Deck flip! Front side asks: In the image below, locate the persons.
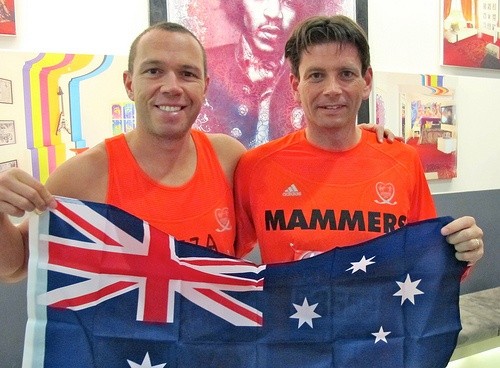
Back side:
[234,14,484,281]
[0,23,248,285]
[192,0,342,150]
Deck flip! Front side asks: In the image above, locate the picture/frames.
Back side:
[148,0,369,150]
[438,0,500,72]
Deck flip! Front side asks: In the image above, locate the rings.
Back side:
[475,238,479,248]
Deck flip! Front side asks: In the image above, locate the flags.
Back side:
[22,196,469,368]
